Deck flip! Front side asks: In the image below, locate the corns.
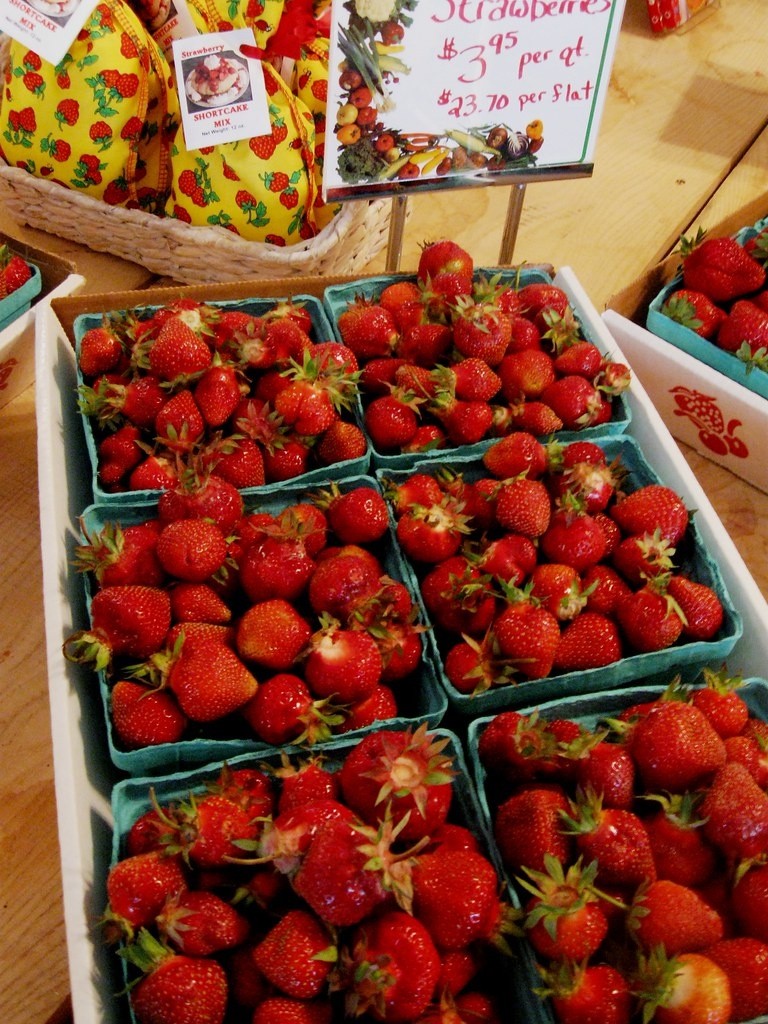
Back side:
[338,54,411,74]
[446,130,501,156]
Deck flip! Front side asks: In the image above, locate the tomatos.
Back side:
[436,158,453,175]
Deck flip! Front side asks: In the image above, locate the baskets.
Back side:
[0,136,392,284]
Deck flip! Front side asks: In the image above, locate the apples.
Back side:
[376,134,394,152]
[397,163,420,179]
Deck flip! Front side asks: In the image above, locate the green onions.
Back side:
[336,17,395,113]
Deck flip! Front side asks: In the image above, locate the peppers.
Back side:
[487,155,506,170]
[526,120,544,154]
[335,21,404,145]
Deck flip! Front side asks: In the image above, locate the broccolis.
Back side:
[342,0,418,39]
[336,137,377,184]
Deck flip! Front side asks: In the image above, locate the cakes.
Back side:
[186,53,248,104]
[28,0,78,15]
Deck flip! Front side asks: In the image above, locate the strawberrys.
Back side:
[0,241,32,301]
[71,225,768,1024]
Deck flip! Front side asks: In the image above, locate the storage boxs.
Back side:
[1,193,768,1024]
